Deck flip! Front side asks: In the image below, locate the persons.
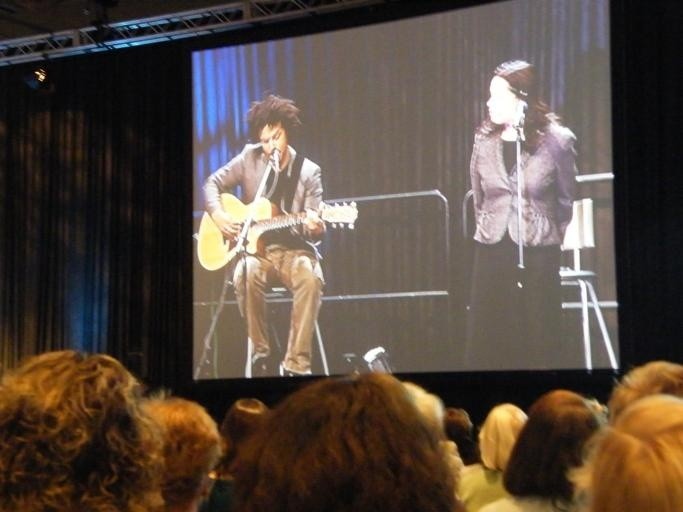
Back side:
[459,57,578,370]
[446,362,683,511]
[0,347,470,512]
[199,92,327,377]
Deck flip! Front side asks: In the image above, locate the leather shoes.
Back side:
[251,353,301,377]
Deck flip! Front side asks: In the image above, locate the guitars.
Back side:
[198,193,358,271]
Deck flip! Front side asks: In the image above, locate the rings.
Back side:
[224,224,231,232]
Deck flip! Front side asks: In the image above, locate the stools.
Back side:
[558,271,618,369]
[245,284,331,378]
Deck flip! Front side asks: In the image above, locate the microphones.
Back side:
[268,141,281,162]
[512,100,529,126]
